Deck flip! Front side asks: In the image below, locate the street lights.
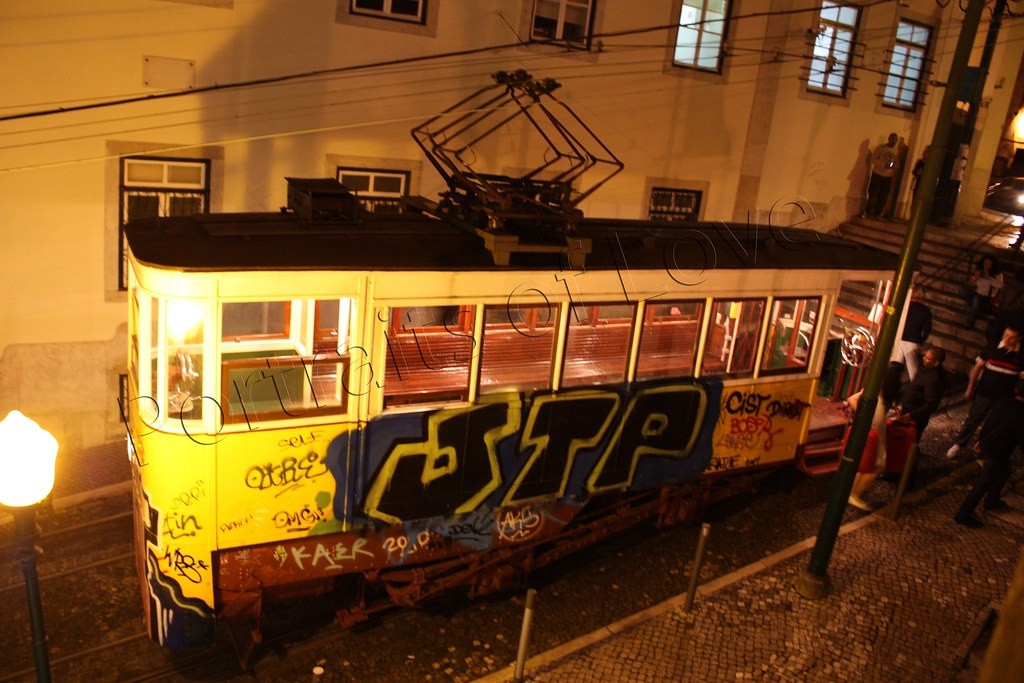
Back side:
[1,411,63,682]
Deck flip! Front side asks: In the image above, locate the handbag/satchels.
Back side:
[884,419,916,474]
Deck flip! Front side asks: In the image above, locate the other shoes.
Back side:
[984,499,1007,511]
[848,495,872,512]
[946,443,961,459]
[954,512,985,529]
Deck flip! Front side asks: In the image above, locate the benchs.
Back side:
[384,318,726,395]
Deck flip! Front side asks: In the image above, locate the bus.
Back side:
[116,170,926,678]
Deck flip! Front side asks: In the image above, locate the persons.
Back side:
[911,145,930,223]
[947,254,1024,525]
[1008,223,1024,250]
[843,284,946,513]
[861,133,899,221]
